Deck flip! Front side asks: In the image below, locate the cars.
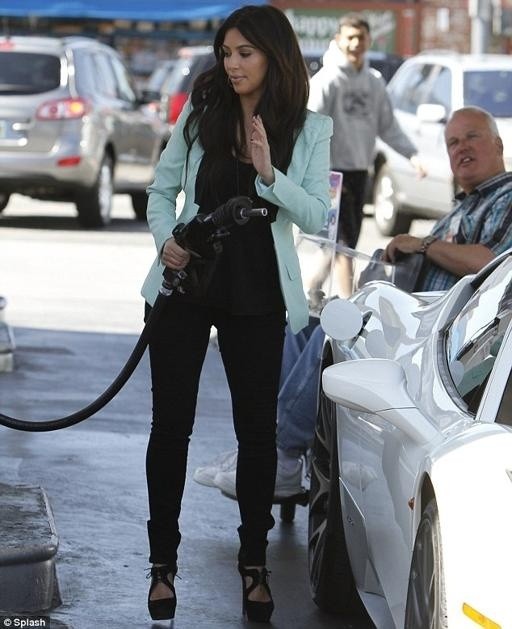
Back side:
[372,48,512,237]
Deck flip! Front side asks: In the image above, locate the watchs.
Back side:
[419,235,437,253]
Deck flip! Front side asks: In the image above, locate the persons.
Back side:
[139,5,333,623]
[308,12,429,313]
[193,107,510,504]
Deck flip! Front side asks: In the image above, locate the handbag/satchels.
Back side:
[357,249,423,293]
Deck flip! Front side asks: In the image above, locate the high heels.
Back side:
[147,559,179,620]
[237,561,275,621]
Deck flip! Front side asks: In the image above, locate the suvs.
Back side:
[159,45,217,125]
[0,33,173,226]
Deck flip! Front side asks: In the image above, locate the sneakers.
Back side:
[214,451,301,499]
[193,448,241,486]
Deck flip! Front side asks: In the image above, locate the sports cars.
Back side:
[304,247,512,629]
